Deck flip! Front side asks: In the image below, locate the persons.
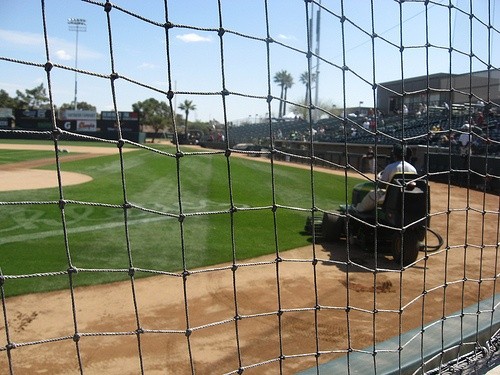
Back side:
[177,81,500,217]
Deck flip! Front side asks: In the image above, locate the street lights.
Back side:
[67,17,86,109]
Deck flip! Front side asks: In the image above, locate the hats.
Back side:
[391,143,407,159]
